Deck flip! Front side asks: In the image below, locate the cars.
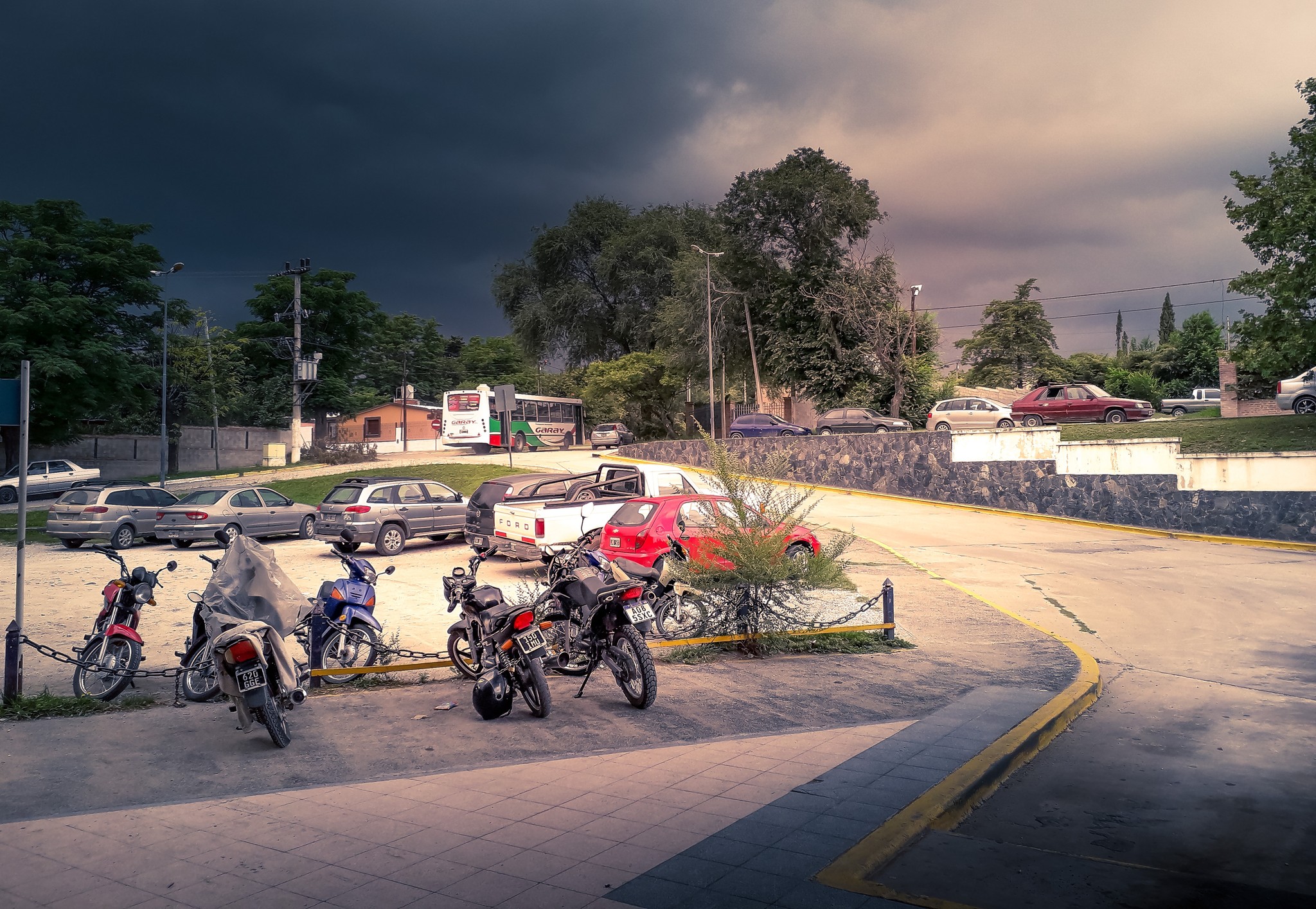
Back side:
[1276,364,1316,415]
[816,408,913,436]
[44,484,186,549]
[0,458,106,502]
[598,494,823,585]
[925,397,1019,431]
[591,423,635,451]
[1011,383,1156,427]
[317,477,479,558]
[728,414,813,438]
[460,471,600,559]
[153,488,320,546]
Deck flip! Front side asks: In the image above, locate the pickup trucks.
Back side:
[493,461,759,558]
[1162,387,1224,418]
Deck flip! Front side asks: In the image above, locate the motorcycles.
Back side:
[291,550,398,685]
[191,525,315,747]
[610,521,711,641]
[524,531,663,713]
[440,544,553,722]
[69,542,177,701]
[177,550,247,703]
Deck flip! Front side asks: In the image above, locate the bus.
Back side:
[439,388,588,448]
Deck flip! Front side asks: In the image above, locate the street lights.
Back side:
[151,261,187,482]
[690,243,725,439]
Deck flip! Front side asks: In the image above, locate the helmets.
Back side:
[472,667,518,721]
